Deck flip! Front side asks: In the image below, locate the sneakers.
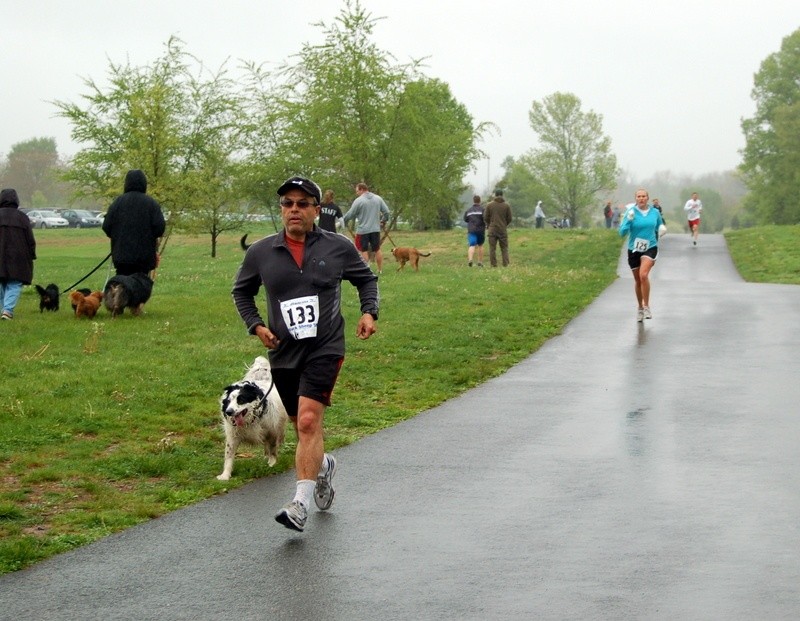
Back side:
[313,454,338,510]
[275,499,310,534]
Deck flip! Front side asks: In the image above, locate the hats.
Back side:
[276,176,321,204]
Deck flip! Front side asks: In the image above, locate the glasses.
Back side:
[280,199,316,209]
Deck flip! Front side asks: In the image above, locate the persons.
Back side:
[318,189,343,233]
[618,188,667,322]
[683,194,703,245]
[231,176,380,532]
[463,189,512,268]
[342,183,390,274]
[0,188,38,320]
[534,201,571,227]
[103,168,166,314]
[605,202,620,230]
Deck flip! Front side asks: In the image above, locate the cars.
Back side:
[18,206,281,230]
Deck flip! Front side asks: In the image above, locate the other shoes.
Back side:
[468,261,474,266]
[377,269,383,273]
[644,306,652,319]
[637,307,644,322]
[693,240,697,246]
[477,262,485,267]
[1,312,14,320]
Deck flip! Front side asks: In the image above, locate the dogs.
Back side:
[216,355,289,480]
[103,272,153,319]
[71,287,103,319]
[34,284,60,314]
[391,247,431,272]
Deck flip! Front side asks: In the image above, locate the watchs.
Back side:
[363,308,379,320]
